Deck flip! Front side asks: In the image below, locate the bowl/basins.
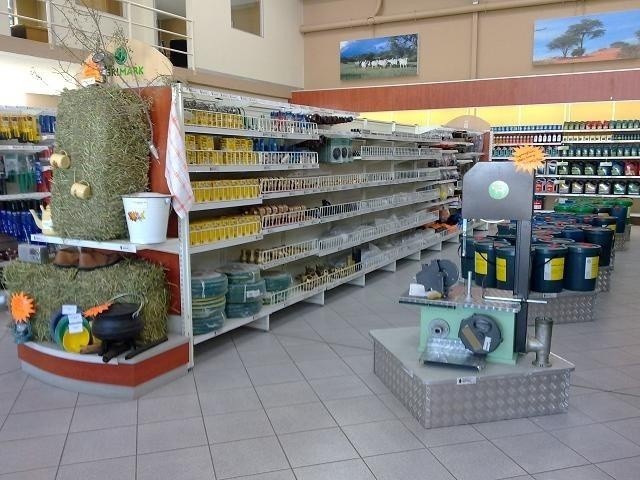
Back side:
[50,310,89,354]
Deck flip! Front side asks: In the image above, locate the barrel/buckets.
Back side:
[120,191,174,244]
[458,161,640,294]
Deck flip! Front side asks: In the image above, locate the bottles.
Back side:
[494,134,562,158]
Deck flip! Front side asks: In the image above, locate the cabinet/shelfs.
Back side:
[481,119,639,219]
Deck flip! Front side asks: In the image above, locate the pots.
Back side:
[93,304,147,352]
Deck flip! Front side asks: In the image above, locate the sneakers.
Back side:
[79,251,121,272]
[54,246,80,268]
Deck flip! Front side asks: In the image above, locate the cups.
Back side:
[50,149,70,168]
[71,180,90,201]
